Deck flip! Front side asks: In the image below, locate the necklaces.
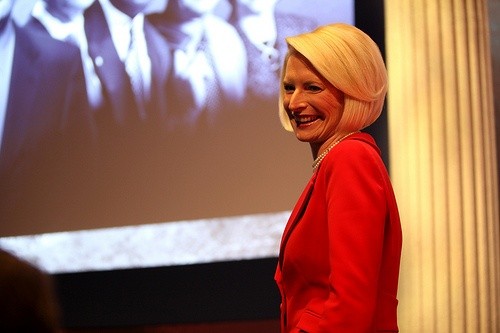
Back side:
[311,130,363,170]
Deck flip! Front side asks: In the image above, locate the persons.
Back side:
[271,20,403,331]
[0,0,280,224]
